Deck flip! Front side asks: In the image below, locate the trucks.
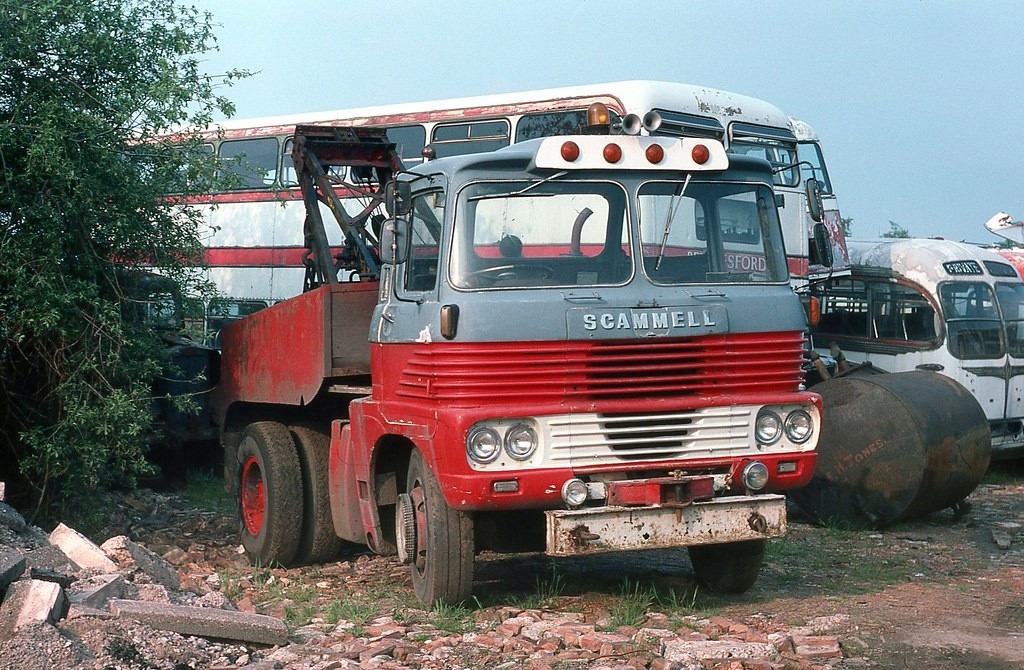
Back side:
[206,103,836,614]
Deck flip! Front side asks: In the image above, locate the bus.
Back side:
[103,80,832,334]
[807,233,1024,455]
